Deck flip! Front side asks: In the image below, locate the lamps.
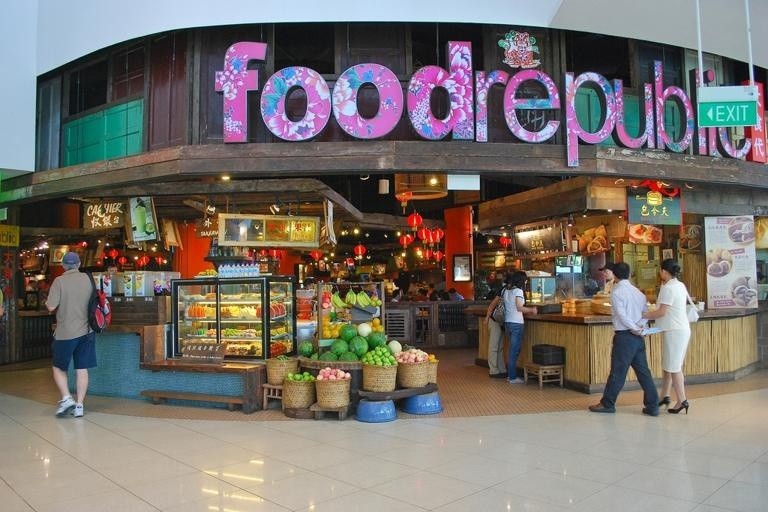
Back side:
[268,192,284,214]
[378,173,389,193]
[205,184,216,216]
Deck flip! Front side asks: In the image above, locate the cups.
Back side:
[133,206,148,234]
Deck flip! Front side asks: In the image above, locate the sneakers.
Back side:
[589,403,616,412]
[71,405,84,416]
[642,407,659,416]
[507,376,525,384]
[55,397,75,417]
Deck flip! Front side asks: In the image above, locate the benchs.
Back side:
[142,390,246,411]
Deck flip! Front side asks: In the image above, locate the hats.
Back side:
[63,252,80,265]
[598,261,615,271]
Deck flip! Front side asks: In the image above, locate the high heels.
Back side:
[659,396,670,410]
[668,400,689,414]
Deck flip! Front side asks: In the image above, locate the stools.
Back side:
[523,362,564,388]
[309,401,352,421]
[263,383,283,411]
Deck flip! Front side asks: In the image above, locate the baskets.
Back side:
[265,357,439,408]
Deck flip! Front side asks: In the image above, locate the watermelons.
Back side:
[298,323,386,361]
[276,354,288,361]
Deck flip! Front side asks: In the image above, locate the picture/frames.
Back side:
[453,254,472,283]
[49,244,69,266]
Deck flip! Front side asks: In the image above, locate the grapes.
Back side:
[402,343,414,352]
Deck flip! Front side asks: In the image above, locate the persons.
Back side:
[46,252,93,416]
[483,272,514,378]
[589,262,660,417]
[598,262,618,295]
[390,283,464,343]
[501,271,537,384]
[584,276,598,296]
[642,258,691,415]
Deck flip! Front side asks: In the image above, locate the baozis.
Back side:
[755,217,768,248]
[628,223,662,243]
[732,277,756,306]
[677,225,702,249]
[706,247,733,275]
[728,218,755,243]
[577,225,611,253]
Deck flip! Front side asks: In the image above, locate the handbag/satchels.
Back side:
[493,301,505,321]
[686,304,699,322]
[88,289,112,333]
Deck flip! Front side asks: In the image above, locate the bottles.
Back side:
[217,263,259,278]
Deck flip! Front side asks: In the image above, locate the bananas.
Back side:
[355,291,371,307]
[345,289,357,305]
[330,291,346,307]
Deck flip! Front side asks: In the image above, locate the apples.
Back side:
[393,349,428,364]
[360,346,397,366]
[316,367,349,380]
[286,372,315,381]
[370,296,381,307]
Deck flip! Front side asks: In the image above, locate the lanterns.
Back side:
[500,236,511,250]
[395,191,444,263]
[354,245,366,259]
[310,249,322,261]
[109,249,164,267]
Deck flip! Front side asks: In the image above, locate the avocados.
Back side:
[145,223,155,236]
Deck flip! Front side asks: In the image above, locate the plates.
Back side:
[726,216,754,245]
[730,276,757,307]
[706,246,733,278]
[680,225,702,249]
[629,224,663,244]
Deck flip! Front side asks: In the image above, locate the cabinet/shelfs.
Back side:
[171,277,296,361]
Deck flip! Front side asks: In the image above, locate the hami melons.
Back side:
[387,340,402,355]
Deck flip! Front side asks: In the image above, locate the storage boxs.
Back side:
[532,344,564,365]
